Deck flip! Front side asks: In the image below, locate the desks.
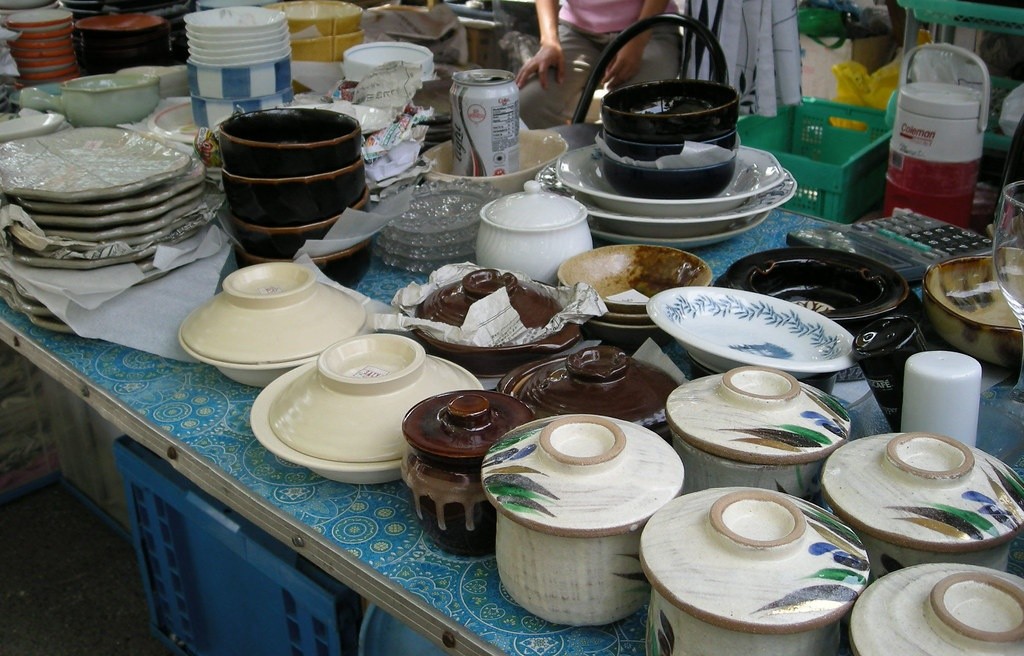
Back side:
[0,210,1024,656]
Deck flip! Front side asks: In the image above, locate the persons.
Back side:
[516,0,681,129]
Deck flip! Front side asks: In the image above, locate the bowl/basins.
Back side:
[177,244,1024,655]
[6,1,440,285]
[921,255,1024,370]
[420,79,798,252]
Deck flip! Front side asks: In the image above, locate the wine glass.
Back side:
[981,181,1024,424]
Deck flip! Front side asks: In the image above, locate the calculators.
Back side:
[785,213,995,287]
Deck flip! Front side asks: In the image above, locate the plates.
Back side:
[1,127,211,335]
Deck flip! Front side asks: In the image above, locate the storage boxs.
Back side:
[897,0,1024,36]
[887,74,1023,152]
[736,95,892,225]
[113,434,363,655]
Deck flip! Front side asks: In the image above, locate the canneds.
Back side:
[450,69,519,177]
